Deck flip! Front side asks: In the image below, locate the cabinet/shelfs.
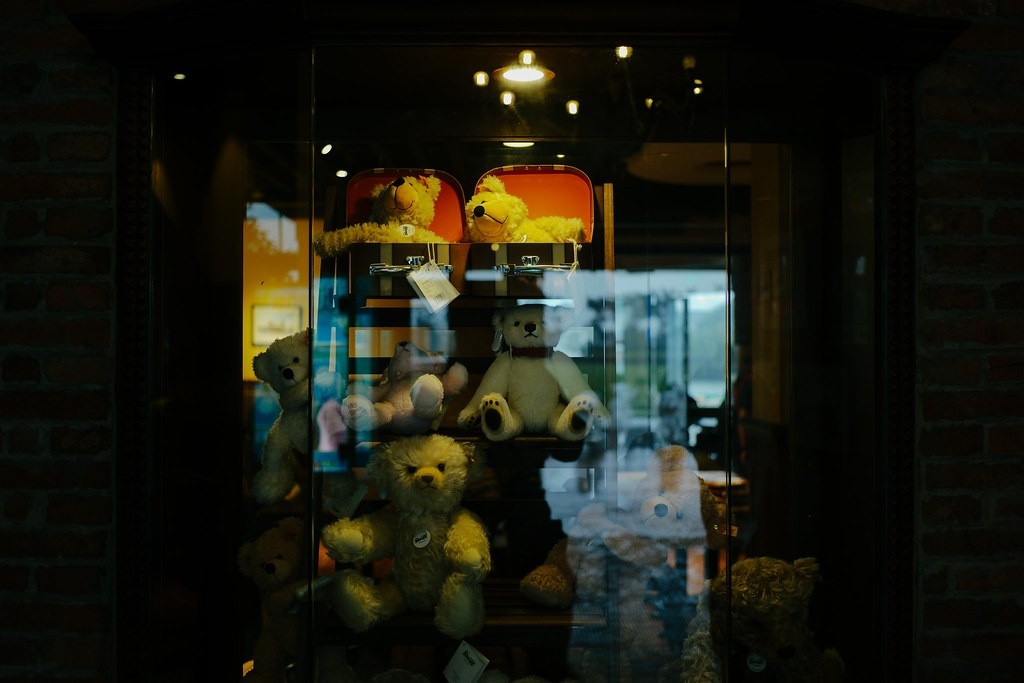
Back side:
[307,159,620,683]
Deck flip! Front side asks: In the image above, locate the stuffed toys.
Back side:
[341,339,469,432]
[458,174,588,244]
[519,445,736,683]
[456,304,613,442]
[235,517,311,683]
[248,327,313,509]
[311,174,452,256]
[322,434,491,639]
[678,556,849,683]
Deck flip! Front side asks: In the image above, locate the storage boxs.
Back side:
[339,165,596,298]
